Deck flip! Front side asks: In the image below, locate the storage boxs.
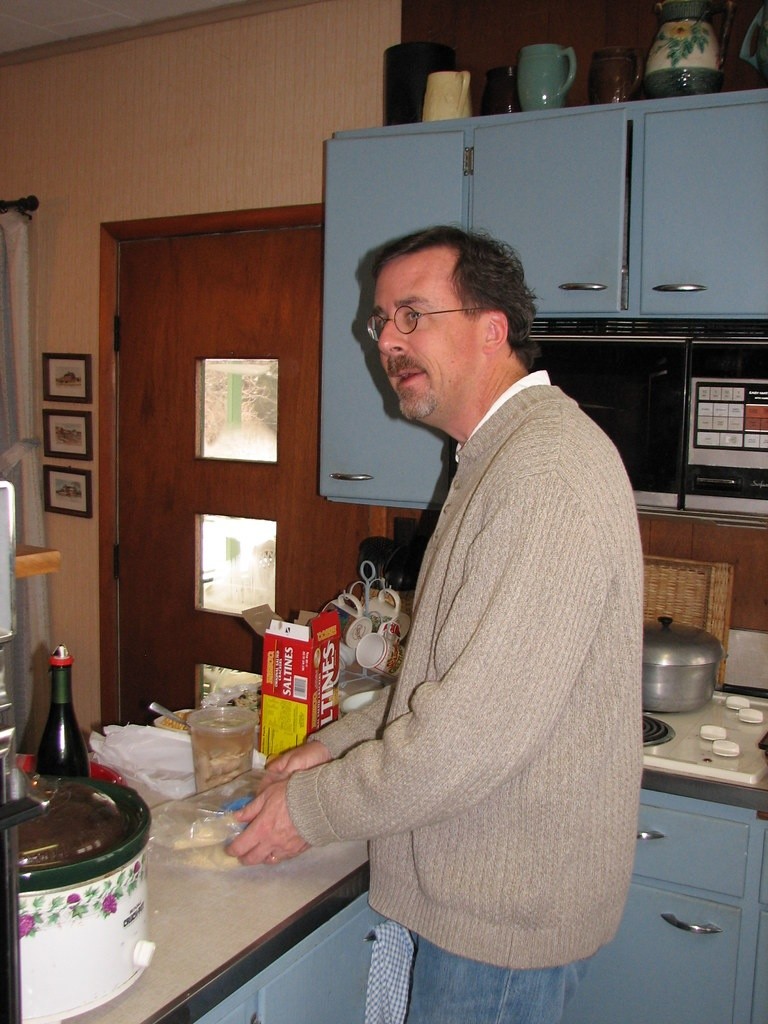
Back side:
[233,599,344,773]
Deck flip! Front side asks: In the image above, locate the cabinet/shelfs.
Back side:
[169,792,768,1024]
[317,85,768,508]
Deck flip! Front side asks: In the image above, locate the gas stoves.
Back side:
[640,627,768,786]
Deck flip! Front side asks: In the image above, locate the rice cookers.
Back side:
[15,773,156,1024]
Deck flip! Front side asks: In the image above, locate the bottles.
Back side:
[34,642,91,778]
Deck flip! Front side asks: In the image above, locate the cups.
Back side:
[321,593,372,648]
[188,707,260,795]
[518,43,578,113]
[363,588,410,641]
[382,40,457,126]
[356,622,405,681]
[478,63,520,116]
[587,46,647,106]
[421,70,474,123]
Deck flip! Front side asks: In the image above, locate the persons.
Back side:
[224,226,644,1024]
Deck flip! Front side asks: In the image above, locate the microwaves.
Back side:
[445,316,768,516]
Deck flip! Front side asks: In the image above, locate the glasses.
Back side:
[366,304,492,341]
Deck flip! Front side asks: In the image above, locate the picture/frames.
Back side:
[43,464,94,519]
[40,407,94,463]
[40,350,93,403]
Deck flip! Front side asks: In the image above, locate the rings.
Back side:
[271,852,282,864]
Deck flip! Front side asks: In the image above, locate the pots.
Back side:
[643,615,728,713]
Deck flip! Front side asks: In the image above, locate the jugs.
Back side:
[641,0,734,100]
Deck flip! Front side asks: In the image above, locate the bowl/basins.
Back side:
[340,688,375,718]
[154,708,194,734]
